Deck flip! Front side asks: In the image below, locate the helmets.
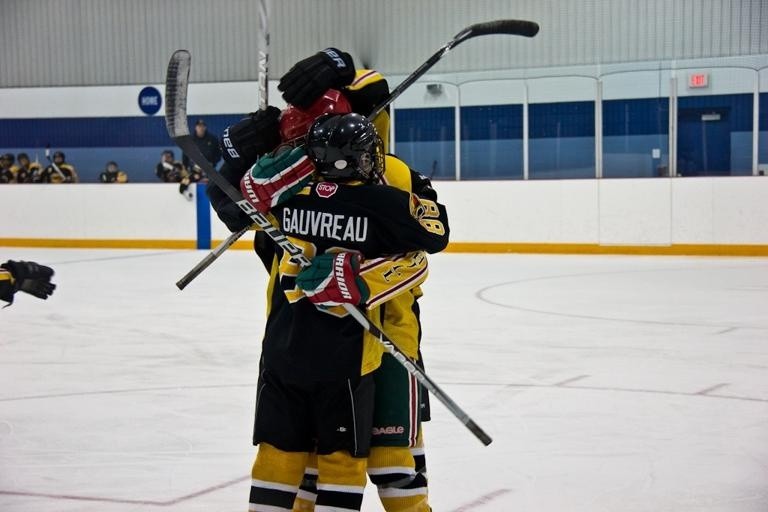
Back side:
[5,153,15,166]
[279,88,385,184]
[54,151,65,164]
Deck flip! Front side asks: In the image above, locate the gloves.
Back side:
[1,259,55,301]
[295,253,369,309]
[220,106,317,211]
[278,48,355,111]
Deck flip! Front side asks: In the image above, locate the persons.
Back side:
[38,151,80,182]
[201,109,450,512]
[181,118,221,184]
[215,46,438,511]
[0,256,57,308]
[0,152,20,183]
[17,153,43,182]
[154,150,184,182]
[103,162,128,183]
